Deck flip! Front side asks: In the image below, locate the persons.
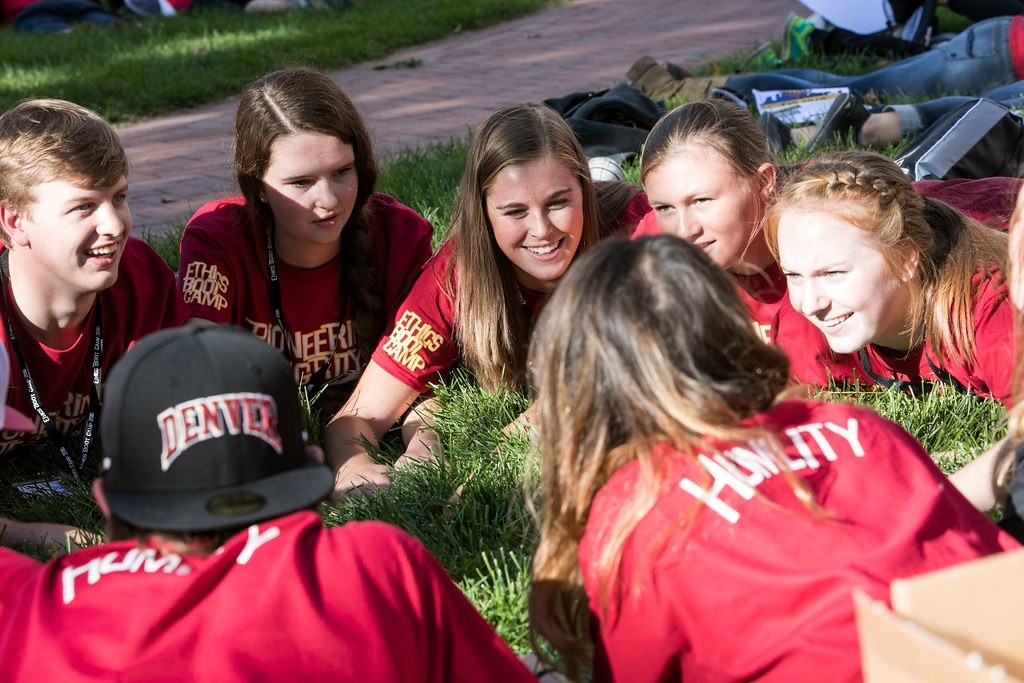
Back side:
[1,320,544,683]
[627,98,828,395]
[766,143,1024,525]
[2,97,194,565]
[513,231,1024,683]
[323,102,655,506]
[179,67,446,504]
[507,0,1024,183]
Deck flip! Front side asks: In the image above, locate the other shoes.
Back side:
[759,113,791,157]
[805,94,867,154]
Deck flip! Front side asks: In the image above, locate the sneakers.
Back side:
[781,9,816,63]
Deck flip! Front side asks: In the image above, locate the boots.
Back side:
[626,56,731,106]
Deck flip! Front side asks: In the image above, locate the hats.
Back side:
[98,320,337,530]
